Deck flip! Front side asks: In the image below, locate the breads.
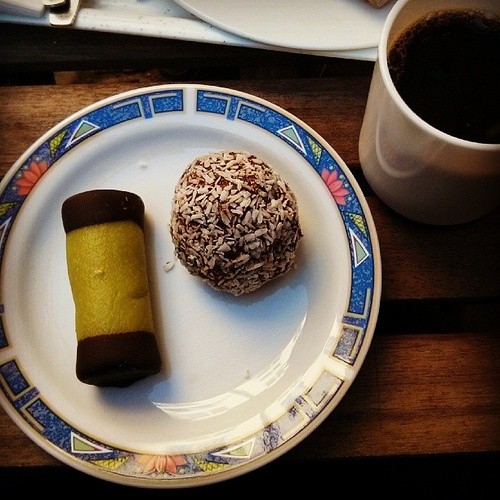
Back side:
[62,189,161,387]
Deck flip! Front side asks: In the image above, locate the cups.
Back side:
[357,0,500,225]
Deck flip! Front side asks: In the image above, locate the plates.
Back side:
[0,85,379,487]
[173,0,397,50]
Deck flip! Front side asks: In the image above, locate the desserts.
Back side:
[169,151,304,297]
